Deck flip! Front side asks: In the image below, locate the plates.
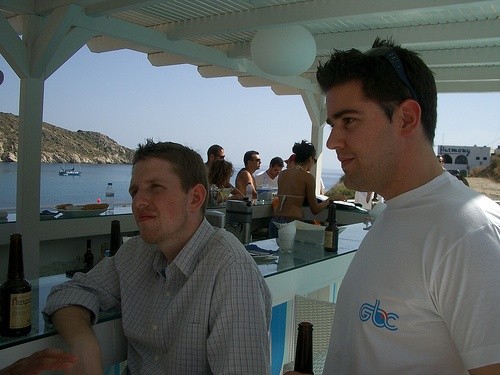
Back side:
[334,201,355,207]
[55,208,107,213]
[244,244,280,258]
[40,210,59,217]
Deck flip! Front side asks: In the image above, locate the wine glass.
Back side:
[216,188,234,205]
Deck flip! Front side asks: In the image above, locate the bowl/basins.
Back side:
[272,220,346,243]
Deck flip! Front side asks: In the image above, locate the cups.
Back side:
[66,262,82,279]
[275,234,295,253]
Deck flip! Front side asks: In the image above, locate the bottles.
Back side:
[211,184,218,208]
[323,204,339,252]
[245,182,253,202]
[294,321,316,375]
[0,233,32,337]
[371,192,379,210]
[102,240,110,258]
[109,219,121,257]
[256,185,263,204]
[83,239,94,273]
[106,183,114,210]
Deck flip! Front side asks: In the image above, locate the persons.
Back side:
[316,34,500,375]
[206,141,347,239]
[0,347,80,375]
[41,138,272,375]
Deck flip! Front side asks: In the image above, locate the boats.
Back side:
[58,165,81,176]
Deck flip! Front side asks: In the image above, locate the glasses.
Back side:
[215,155,225,159]
[312,157,317,163]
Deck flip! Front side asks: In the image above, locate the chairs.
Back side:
[283,293,335,375]
